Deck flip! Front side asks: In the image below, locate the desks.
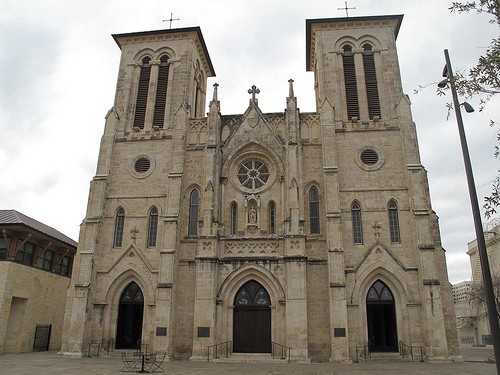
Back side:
[133,351,154,373]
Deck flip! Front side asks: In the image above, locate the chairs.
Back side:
[119,351,135,373]
[142,353,157,373]
[152,352,167,373]
